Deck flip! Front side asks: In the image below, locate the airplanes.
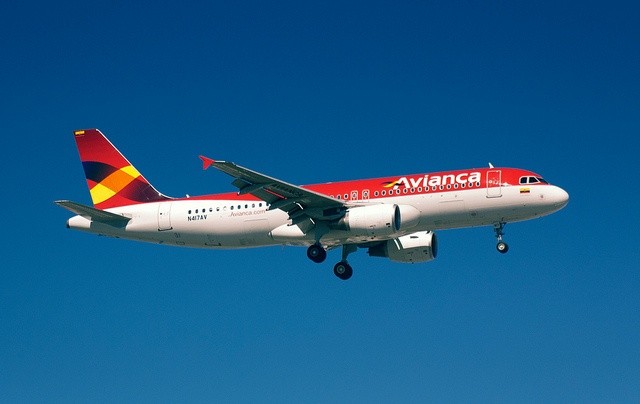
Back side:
[53,129,569,280]
